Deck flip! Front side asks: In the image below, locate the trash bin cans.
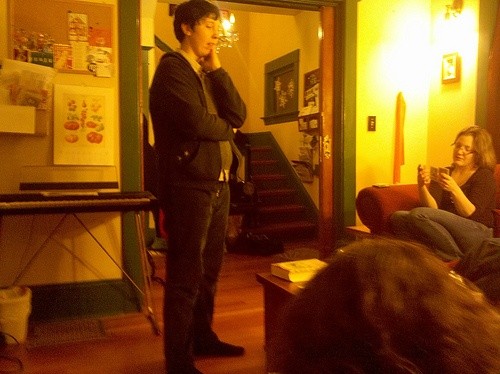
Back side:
[0,286,31,345]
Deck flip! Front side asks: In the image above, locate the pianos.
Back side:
[1,190,160,333]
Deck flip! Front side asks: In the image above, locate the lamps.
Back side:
[214,12,239,54]
[444,0,464,19]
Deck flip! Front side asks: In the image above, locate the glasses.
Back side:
[451,143,475,155]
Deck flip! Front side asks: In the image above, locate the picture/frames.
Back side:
[53,84,115,168]
[441,51,462,83]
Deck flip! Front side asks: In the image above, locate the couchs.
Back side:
[355,165,500,268]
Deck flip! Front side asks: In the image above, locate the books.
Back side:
[270,258,328,282]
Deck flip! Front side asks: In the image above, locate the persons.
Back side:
[148,0,247,374]
[388,125,495,258]
[265,238,500,374]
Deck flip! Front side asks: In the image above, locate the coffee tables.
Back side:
[255,271,309,351]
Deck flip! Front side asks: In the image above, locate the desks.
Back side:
[0,191,162,336]
